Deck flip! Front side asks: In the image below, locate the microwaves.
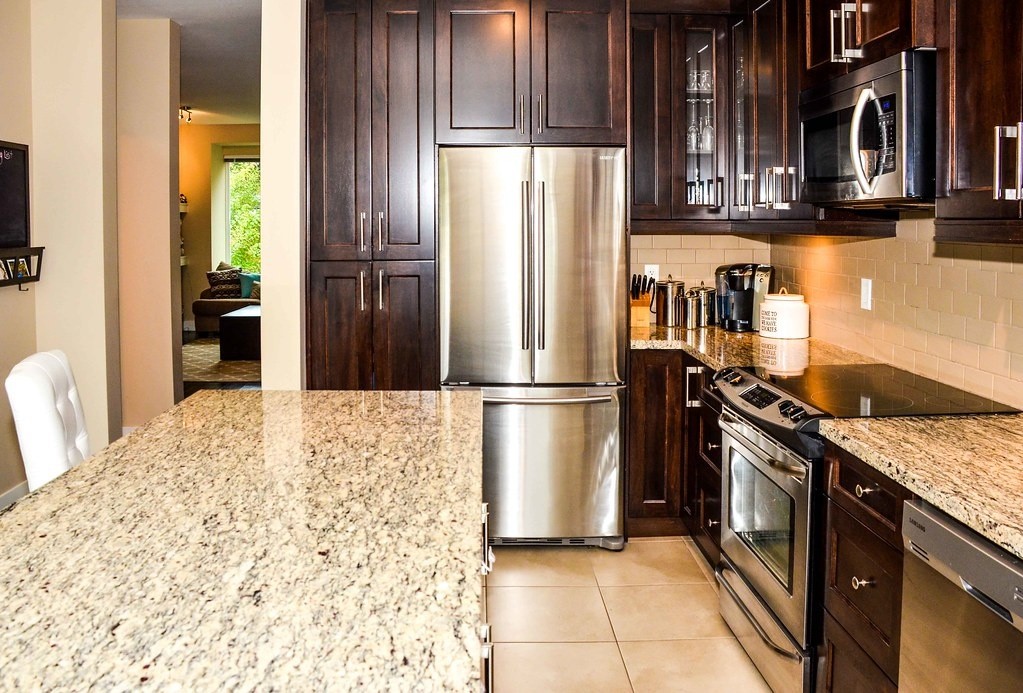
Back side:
[797,50,936,214]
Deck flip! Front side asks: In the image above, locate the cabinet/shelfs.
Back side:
[934,0,1023,246]
[815,447,905,693]
[730,0,900,238]
[303,1,438,393]
[434,2,628,149]
[684,350,723,575]
[629,348,688,536]
[666,1,731,233]
[802,1,929,82]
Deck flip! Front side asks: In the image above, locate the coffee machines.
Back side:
[715,263,776,332]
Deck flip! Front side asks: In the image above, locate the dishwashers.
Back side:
[898,497,1022,693]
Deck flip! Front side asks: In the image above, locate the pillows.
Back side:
[216,261,232,271]
[250,280,260,299]
[239,273,260,298]
[206,267,242,298]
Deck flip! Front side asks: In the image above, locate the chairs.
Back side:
[5,350,91,492]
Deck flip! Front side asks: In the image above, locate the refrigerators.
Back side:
[438,146,625,552]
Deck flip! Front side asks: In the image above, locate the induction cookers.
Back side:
[713,363,1022,456]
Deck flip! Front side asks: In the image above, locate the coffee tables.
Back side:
[219,305,262,361]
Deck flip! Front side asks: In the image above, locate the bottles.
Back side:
[656,273,715,331]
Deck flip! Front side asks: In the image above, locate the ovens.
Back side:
[714,404,821,693]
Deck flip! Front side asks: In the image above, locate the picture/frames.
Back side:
[0,261,8,280]
[7,260,30,278]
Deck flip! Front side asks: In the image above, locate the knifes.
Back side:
[631,273,653,300]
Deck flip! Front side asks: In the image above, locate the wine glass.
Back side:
[685,69,714,151]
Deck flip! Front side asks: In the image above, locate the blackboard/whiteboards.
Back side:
[0,140,31,279]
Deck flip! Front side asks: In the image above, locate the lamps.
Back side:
[179,109,183,119]
[186,111,192,123]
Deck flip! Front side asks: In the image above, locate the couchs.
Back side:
[191,288,261,335]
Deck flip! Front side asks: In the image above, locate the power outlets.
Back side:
[860,396,871,417]
[644,264,662,287]
[860,278,872,311]
